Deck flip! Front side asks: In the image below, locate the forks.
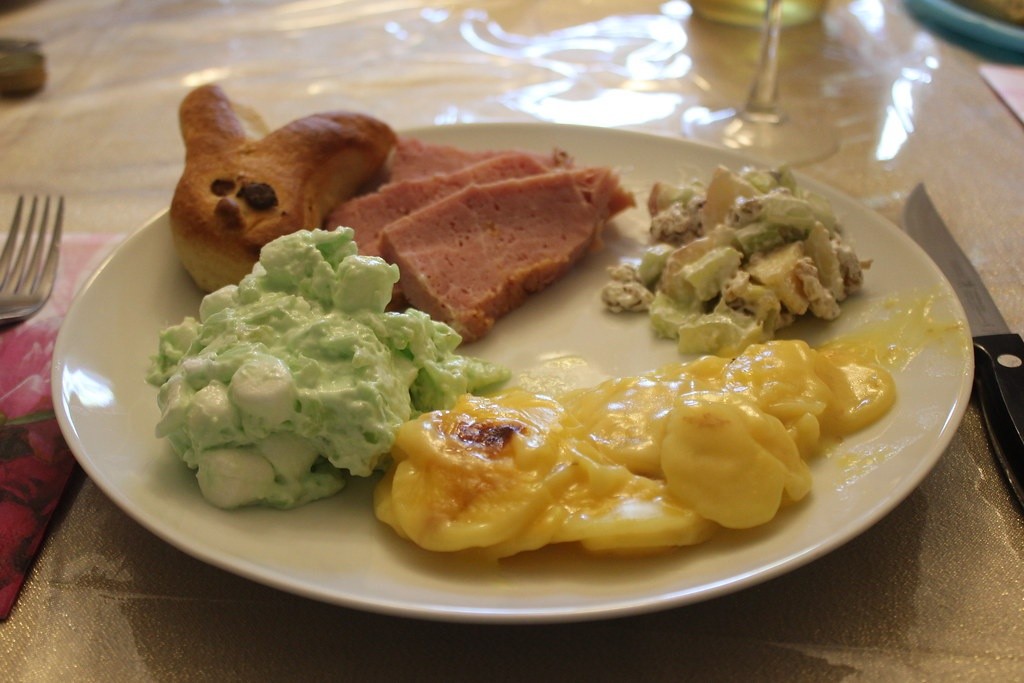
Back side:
[0,194,67,318]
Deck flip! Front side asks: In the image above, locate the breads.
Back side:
[170,82,399,292]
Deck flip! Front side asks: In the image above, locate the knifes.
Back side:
[904,179,1024,518]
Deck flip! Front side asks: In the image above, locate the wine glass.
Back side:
[681,0,841,163]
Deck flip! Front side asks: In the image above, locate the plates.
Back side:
[908,0,1024,56]
[47,122,974,623]
